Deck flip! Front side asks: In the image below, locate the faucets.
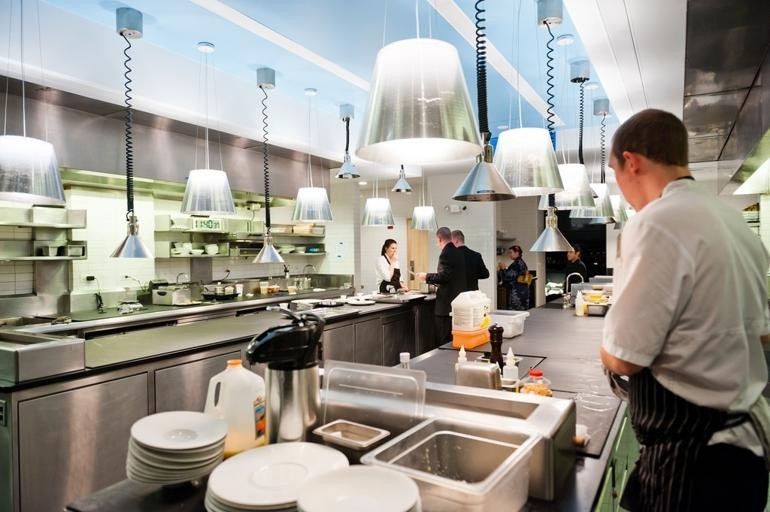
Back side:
[176,272,189,285]
[566,273,584,294]
[302,264,317,278]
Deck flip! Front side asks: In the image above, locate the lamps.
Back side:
[359,1,630,253]
[0,0,66,207]
[109,32,153,259]
[290,96,333,221]
[334,118,362,180]
[253,84,284,264]
[180,49,238,216]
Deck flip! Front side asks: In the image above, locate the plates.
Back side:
[295,299,376,307]
[125,411,423,512]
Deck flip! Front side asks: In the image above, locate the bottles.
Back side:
[574,291,584,316]
[452,345,468,386]
[487,323,552,397]
[398,352,412,371]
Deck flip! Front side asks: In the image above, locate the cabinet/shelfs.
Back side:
[382,309,419,369]
[155,216,328,256]
[420,308,435,355]
[1,207,89,261]
[13,372,148,508]
[148,343,247,413]
[323,315,382,366]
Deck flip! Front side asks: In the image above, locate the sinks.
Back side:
[374,418,542,512]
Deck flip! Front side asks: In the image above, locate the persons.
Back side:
[598,108,770,512]
[415,227,467,345]
[451,230,490,290]
[375,239,403,292]
[500,245,531,311]
[564,243,587,292]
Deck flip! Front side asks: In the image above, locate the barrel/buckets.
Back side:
[204,358,270,456]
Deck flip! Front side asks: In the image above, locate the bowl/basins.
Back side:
[294,246,306,253]
[268,286,279,293]
[171,240,219,255]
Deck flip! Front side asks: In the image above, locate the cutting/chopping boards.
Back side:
[375,295,426,305]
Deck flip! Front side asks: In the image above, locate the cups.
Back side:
[259,281,270,295]
[340,290,379,299]
[235,284,244,296]
[288,286,296,294]
